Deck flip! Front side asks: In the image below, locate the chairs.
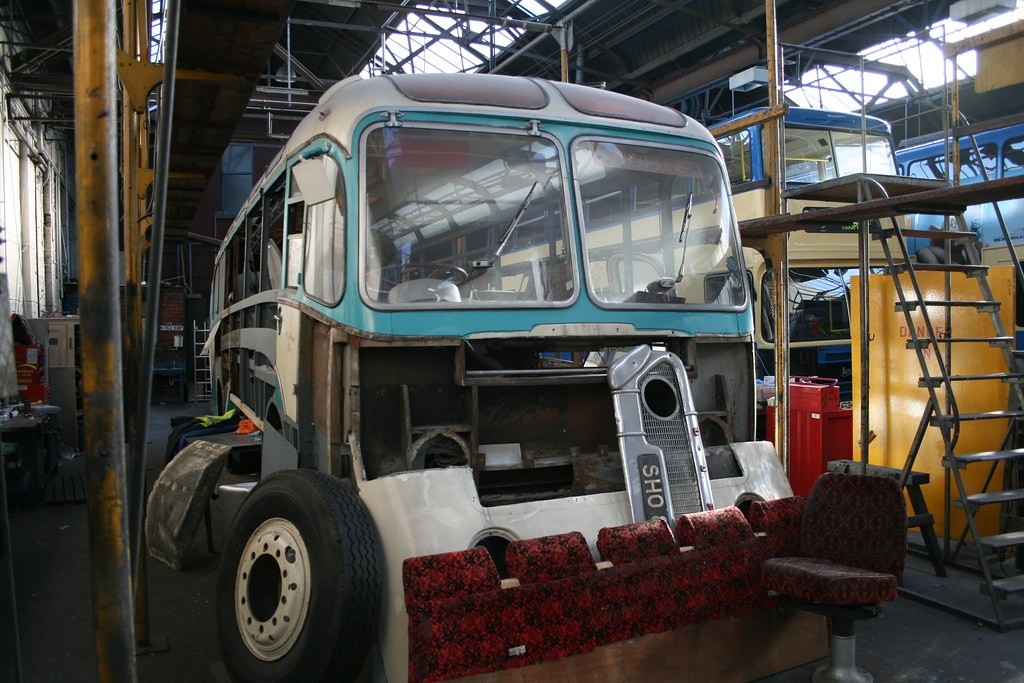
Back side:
[764,472,908,683]
[369,228,402,303]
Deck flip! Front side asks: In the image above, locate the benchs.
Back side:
[828,459,947,578]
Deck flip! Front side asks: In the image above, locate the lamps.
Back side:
[275,63,297,83]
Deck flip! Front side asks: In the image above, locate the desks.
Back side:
[184,432,262,552]
[0,411,57,518]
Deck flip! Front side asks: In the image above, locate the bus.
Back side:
[356,106,912,400]
[1,72,914,682]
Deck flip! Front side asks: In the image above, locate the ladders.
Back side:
[193,320,214,413]
